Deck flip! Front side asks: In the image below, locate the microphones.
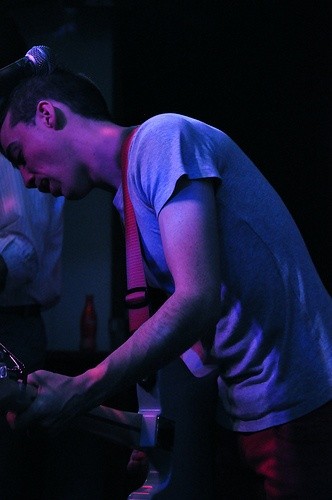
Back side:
[0,44,52,89]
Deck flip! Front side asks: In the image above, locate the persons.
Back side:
[0,69,332,500]
[0,152,68,374]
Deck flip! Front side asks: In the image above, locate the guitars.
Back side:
[0,344,201,500]
